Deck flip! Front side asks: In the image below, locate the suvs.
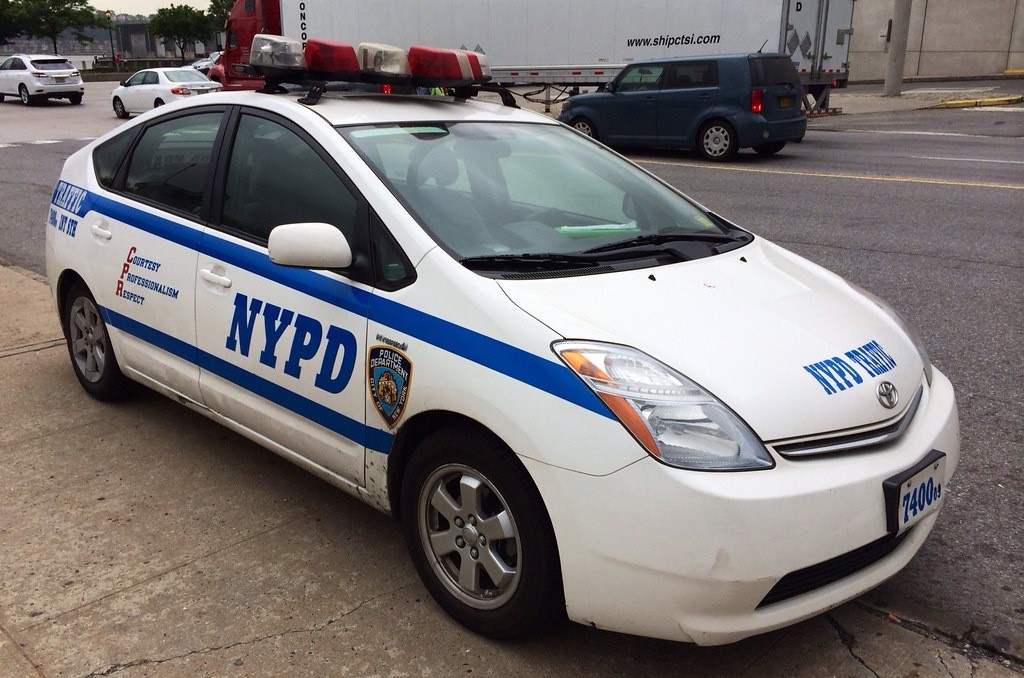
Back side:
[558,52,807,163]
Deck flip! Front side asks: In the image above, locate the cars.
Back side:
[45,34,960,646]
[0,52,85,105]
[192,51,225,75]
[111,67,223,119]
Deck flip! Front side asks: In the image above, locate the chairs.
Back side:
[407,146,493,250]
[247,144,338,235]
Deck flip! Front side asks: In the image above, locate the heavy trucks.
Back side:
[207,0,854,119]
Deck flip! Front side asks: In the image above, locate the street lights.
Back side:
[105,9,117,66]
